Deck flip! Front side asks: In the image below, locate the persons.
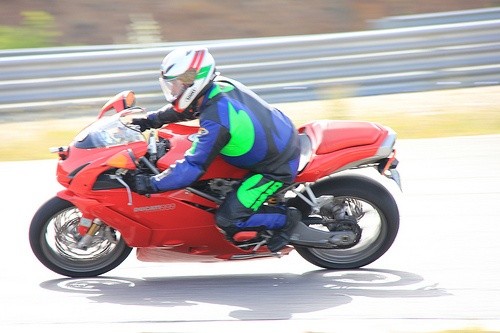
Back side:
[110,44,302,253]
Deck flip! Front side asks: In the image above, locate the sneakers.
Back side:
[267,208,302,253]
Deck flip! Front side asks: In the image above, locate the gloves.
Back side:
[131,116,150,134]
[128,172,157,198]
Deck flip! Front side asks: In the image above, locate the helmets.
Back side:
[161,44,214,112]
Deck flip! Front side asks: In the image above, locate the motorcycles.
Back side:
[28,89,403,277]
